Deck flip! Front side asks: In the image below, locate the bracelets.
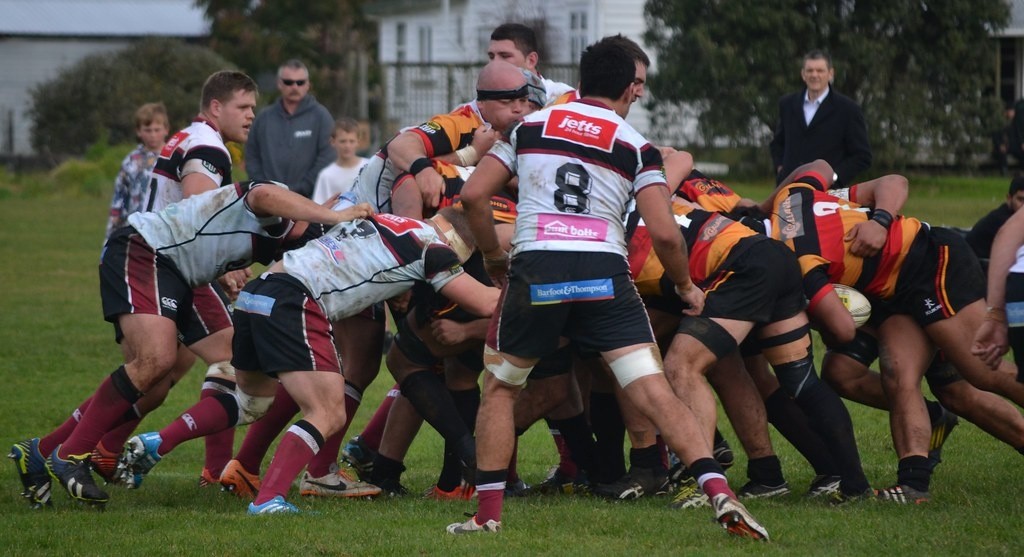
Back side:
[455,145,481,167]
[984,306,1008,325]
[410,158,434,177]
[484,244,500,254]
[873,208,893,231]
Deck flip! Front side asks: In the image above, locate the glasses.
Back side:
[281,78,307,86]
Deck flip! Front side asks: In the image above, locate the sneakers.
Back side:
[116,433,163,489]
[7,438,53,509]
[806,474,840,499]
[928,400,960,474]
[737,481,791,500]
[247,496,322,515]
[300,467,382,497]
[713,493,769,543]
[46,444,110,510]
[88,440,123,484]
[873,485,933,505]
[220,459,263,502]
[446,512,502,535]
[342,435,735,509]
[829,488,877,510]
[199,467,217,487]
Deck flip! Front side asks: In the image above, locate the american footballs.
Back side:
[832,283,871,327]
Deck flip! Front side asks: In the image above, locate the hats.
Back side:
[521,66,547,109]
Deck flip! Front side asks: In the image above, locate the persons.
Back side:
[446,39,771,542]
[769,49,873,191]
[312,119,395,356]
[9,23,1024,517]
[106,103,169,232]
[241,59,335,201]
